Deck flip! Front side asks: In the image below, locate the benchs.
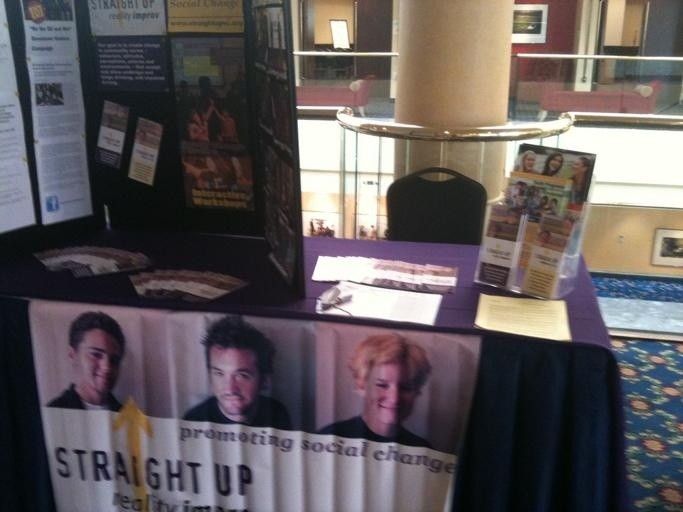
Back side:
[297,74,376,116]
[534,79,664,122]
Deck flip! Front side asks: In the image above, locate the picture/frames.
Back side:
[512,4,550,45]
[651,228,683,268]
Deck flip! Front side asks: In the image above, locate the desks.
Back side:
[0,236,629,511]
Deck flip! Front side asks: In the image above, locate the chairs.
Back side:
[386,164,489,245]
[507,55,519,119]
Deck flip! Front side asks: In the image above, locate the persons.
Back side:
[183,317,292,431]
[507,150,590,214]
[187,97,241,171]
[317,334,433,447]
[46,312,126,411]
